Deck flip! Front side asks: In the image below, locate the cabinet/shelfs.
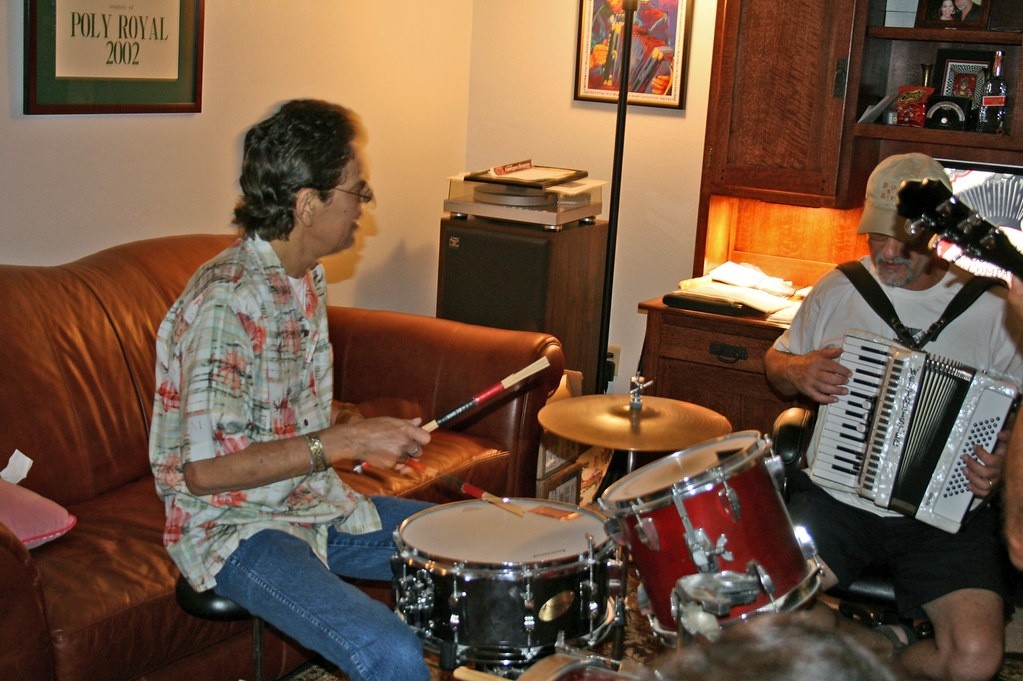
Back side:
[642,314,821,439]
[703,0,1023,211]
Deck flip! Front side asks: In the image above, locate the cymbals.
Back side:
[537,393,734,453]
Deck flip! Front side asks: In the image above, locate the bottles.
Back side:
[976,49,1009,133]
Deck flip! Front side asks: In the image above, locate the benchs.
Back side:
[0,233,561,681]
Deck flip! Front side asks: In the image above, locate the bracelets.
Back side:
[306,432,326,472]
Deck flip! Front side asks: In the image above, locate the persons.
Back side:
[764,152,1023,681]
[149,100,440,681]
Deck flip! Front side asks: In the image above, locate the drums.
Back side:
[598,428,821,649]
[388,502,642,681]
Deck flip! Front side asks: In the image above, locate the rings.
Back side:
[989,478,993,489]
[410,444,420,456]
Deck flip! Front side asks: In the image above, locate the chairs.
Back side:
[774,401,1023,640]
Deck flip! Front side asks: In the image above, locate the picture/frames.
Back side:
[573,0,695,109]
[23,0,204,114]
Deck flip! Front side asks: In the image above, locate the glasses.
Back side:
[332,188,374,202]
[869,232,889,241]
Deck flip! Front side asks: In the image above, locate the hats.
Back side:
[856,153,954,241]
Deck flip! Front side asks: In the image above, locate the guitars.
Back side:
[896,174,1023,282]
